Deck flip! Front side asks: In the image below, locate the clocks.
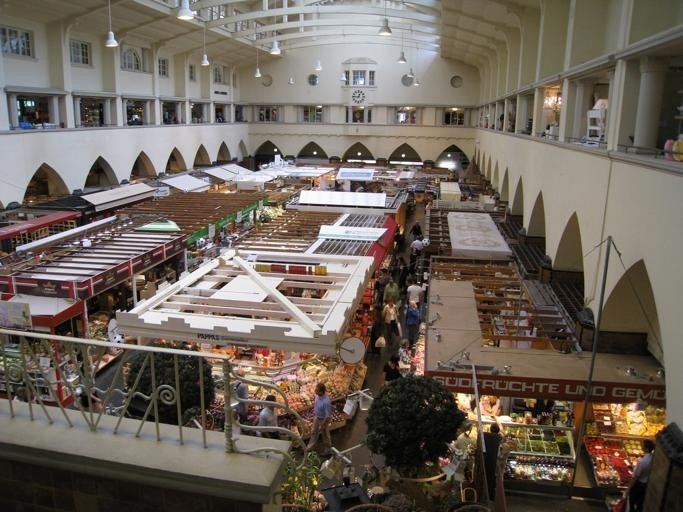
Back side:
[351,90,365,103]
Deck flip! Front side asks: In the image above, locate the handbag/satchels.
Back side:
[374,336,386,348]
[398,351,411,369]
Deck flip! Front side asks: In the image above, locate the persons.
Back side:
[371,219,430,386]
[231,369,249,435]
[77,354,103,413]
[107,307,137,351]
[530,398,554,418]
[480,394,502,417]
[623,439,655,512]
[259,395,282,440]
[301,383,333,458]
[474,416,505,500]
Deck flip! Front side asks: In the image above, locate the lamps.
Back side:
[396,51,405,65]
[176,1,194,20]
[201,54,209,66]
[105,32,118,48]
[377,18,392,36]
[316,60,321,70]
[270,41,280,55]
[255,68,262,78]
